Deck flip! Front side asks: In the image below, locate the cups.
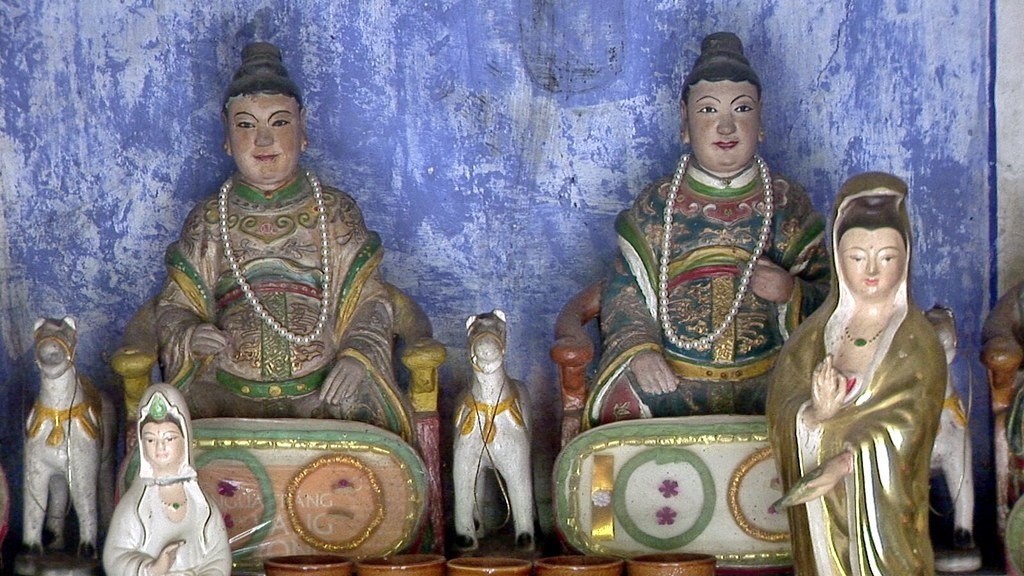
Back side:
[446,556,532,576]
[534,555,626,576]
[356,554,445,576]
[263,554,354,576]
[627,554,716,576]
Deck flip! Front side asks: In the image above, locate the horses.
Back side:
[15,316,120,576]
[921,303,983,573]
[452,308,535,553]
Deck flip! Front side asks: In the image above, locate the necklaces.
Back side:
[843,324,883,347]
[220,168,332,347]
[161,500,187,509]
[659,152,774,350]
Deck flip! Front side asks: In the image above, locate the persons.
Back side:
[102,383,232,576]
[154,44,415,447]
[765,173,948,576]
[584,31,831,430]
